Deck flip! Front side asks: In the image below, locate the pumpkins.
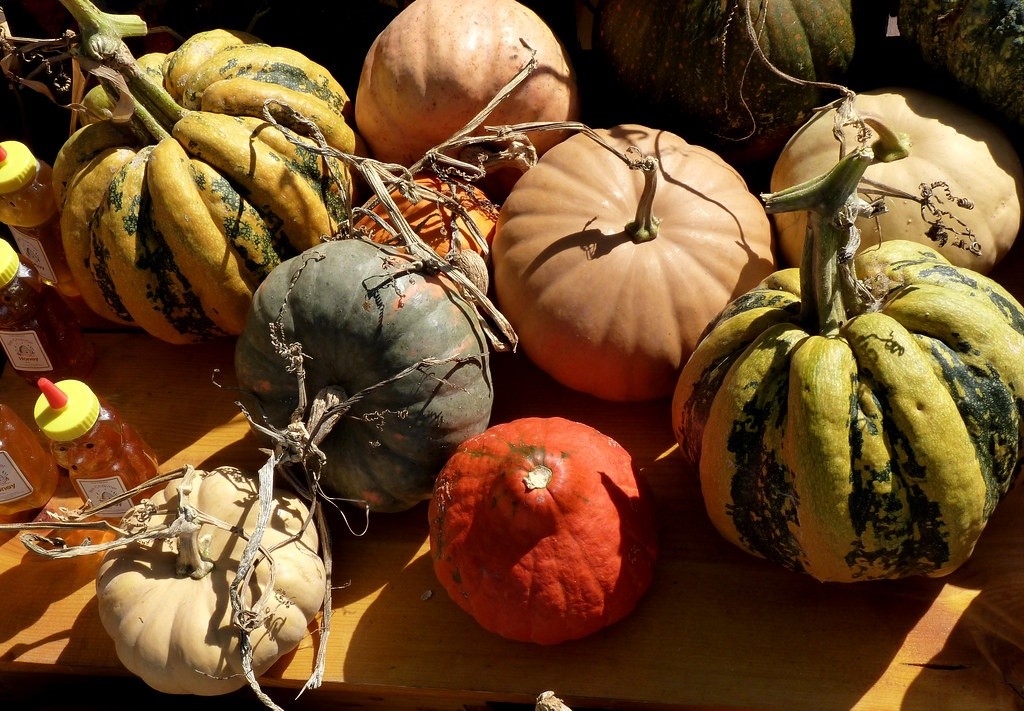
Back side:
[47,0,1024,698]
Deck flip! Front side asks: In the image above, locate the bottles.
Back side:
[0,404,58,515]
[0,140,82,297]
[34,377,161,519]
[0,238,94,381]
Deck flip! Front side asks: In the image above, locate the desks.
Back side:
[0,333,1024,711]
